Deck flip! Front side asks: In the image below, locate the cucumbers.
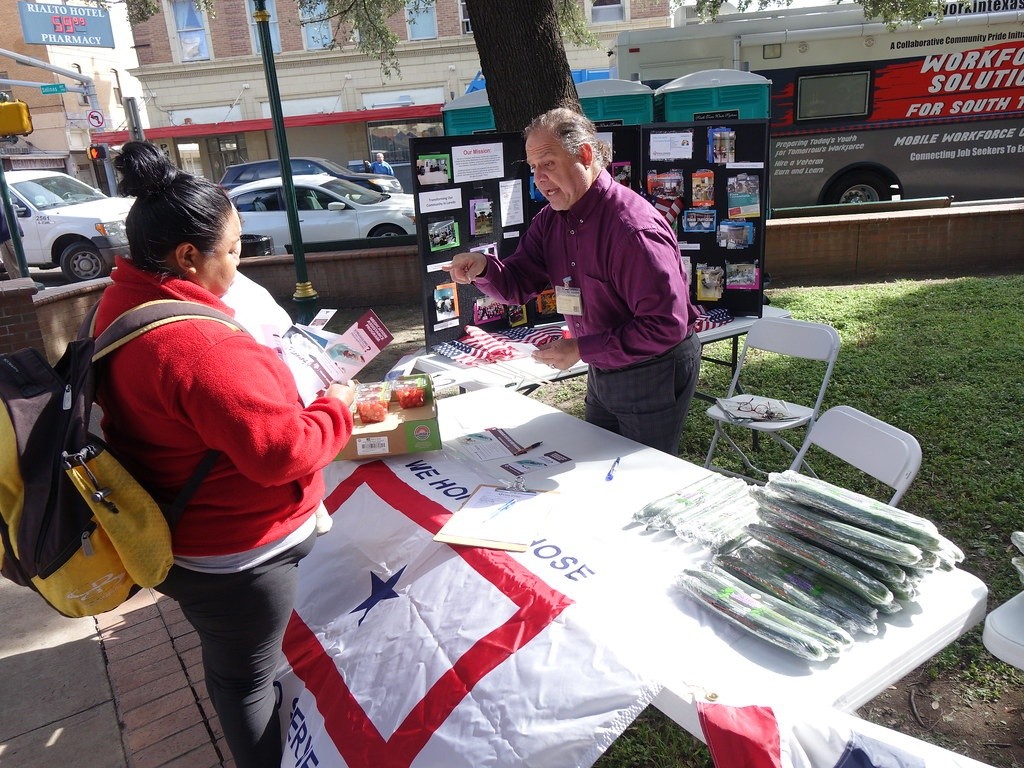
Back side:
[633,470,965,661]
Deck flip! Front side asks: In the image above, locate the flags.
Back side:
[431,323,566,366]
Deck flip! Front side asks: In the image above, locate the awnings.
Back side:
[90,104,442,144]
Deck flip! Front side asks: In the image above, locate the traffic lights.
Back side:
[90,147,106,161]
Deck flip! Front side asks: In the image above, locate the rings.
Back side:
[551,364,556,369]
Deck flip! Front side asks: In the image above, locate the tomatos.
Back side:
[357,398,388,423]
[396,386,424,409]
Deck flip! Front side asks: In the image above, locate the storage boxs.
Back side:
[332,373,443,458]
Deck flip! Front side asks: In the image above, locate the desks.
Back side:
[432,386,992,752]
[415,304,792,450]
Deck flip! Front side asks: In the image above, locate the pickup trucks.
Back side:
[0,169,136,283]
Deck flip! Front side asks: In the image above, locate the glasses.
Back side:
[737,398,771,414]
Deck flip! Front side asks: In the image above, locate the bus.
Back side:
[609,0,1023,208]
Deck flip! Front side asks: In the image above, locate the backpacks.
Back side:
[0,297,255,619]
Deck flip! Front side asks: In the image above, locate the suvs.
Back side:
[228,176,417,257]
[217,155,405,196]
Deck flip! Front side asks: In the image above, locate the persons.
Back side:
[510,308,521,322]
[714,139,734,158]
[478,301,505,321]
[365,152,394,176]
[620,170,630,187]
[728,183,748,194]
[651,186,679,196]
[729,267,748,278]
[440,107,701,457]
[417,163,448,175]
[430,228,455,247]
[87,136,355,767]
[695,182,714,200]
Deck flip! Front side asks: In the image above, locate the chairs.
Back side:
[704,316,842,484]
[304,189,323,209]
[791,405,923,505]
